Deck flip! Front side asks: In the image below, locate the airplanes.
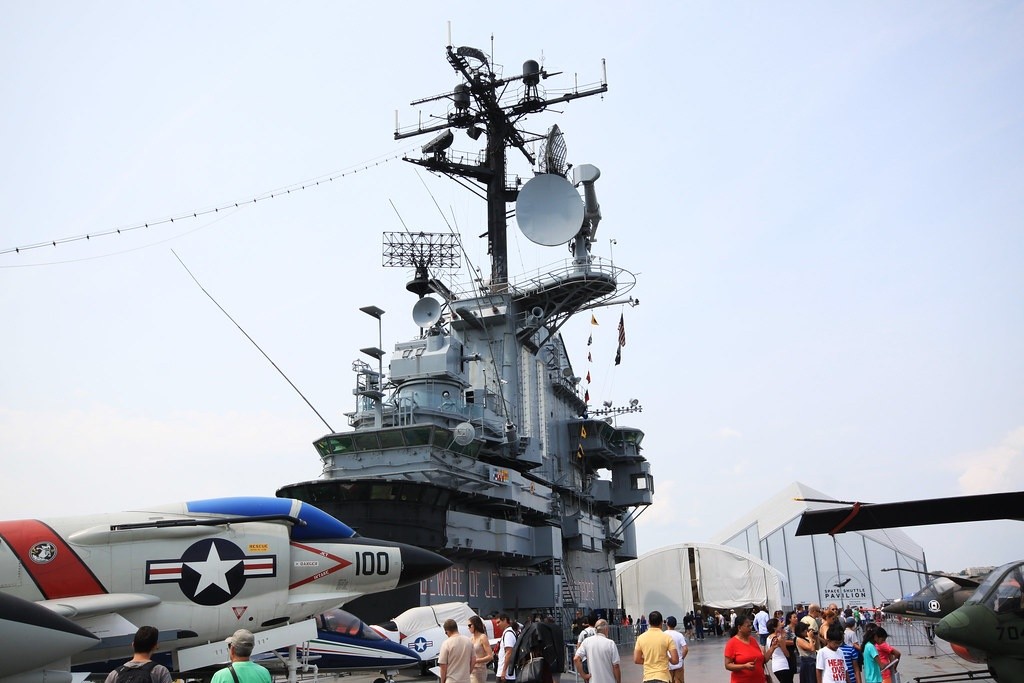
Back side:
[881,559,1024,683]
[0,497,455,683]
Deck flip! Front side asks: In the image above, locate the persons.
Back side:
[684,605,901,683]
[437,619,477,683]
[210,628,272,683]
[634,611,679,683]
[104,626,173,683]
[468,616,494,683]
[923,621,936,646]
[496,613,647,683]
[664,616,688,683]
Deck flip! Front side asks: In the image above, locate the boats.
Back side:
[275,30,658,683]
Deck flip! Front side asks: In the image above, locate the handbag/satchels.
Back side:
[859,612,866,620]
[514,652,544,683]
[764,663,773,683]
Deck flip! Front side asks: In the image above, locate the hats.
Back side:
[730,608,735,613]
[795,622,810,634]
[225,629,255,649]
[846,617,856,625]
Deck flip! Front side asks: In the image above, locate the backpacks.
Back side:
[115,660,159,682]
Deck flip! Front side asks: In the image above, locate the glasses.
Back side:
[832,618,835,621]
[496,620,501,624]
[817,611,822,613]
[741,625,752,627]
[468,624,472,628]
[228,643,231,648]
[832,608,838,611]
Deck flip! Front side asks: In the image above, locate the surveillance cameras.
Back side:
[420,128,451,154]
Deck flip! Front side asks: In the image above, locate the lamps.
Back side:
[629,398,638,408]
[603,400,612,410]
